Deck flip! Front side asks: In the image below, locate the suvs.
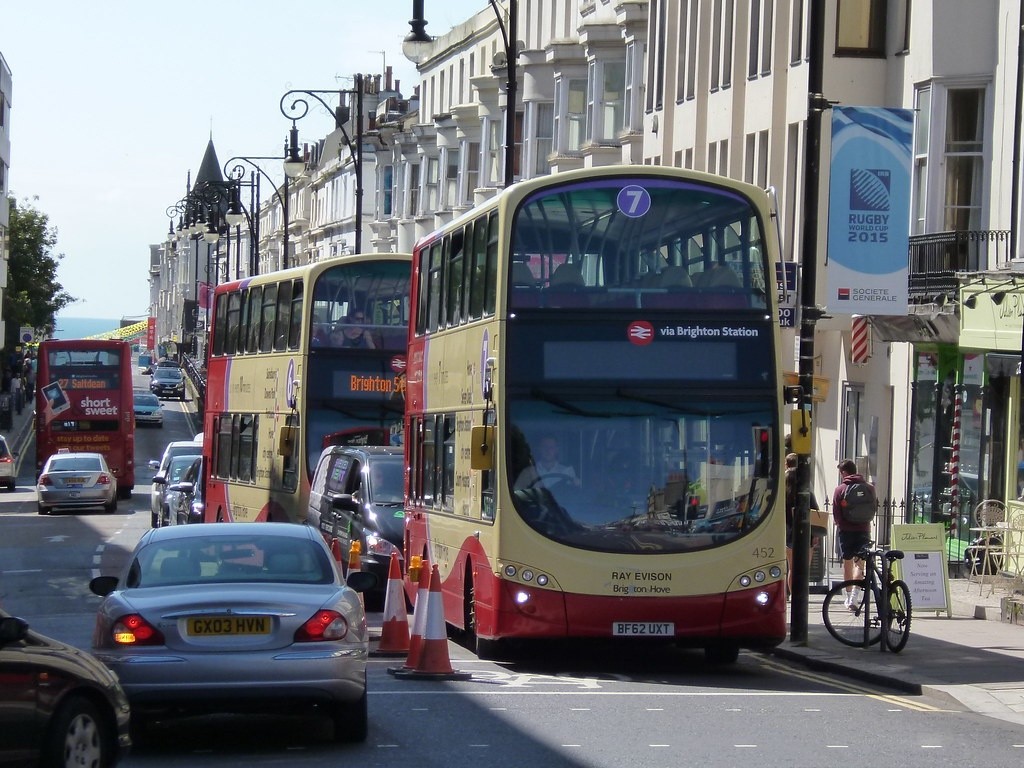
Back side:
[306,443,404,608]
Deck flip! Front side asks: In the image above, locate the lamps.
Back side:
[850,314,873,367]
[932,277,1024,309]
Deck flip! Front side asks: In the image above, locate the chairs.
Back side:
[968,500,1024,600]
[224,319,409,352]
[511,255,749,308]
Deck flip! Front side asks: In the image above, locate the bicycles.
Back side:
[821,539,913,653]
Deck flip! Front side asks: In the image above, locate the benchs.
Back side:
[161,550,304,577]
[945,538,974,580]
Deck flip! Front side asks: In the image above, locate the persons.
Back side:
[353,469,384,497]
[785,434,819,601]
[312,309,376,348]
[513,435,582,490]
[1,368,25,411]
[832,460,878,610]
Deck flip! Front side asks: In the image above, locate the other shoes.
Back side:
[844,597,859,612]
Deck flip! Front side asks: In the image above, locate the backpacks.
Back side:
[842,484,876,524]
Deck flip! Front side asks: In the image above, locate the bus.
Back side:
[33,336,135,500]
[399,159,817,671]
[198,250,485,567]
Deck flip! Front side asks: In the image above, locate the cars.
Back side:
[0,434,20,492]
[146,429,206,528]
[0,606,132,768]
[133,359,186,429]
[89,521,377,744]
[36,448,119,516]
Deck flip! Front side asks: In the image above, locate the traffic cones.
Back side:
[387,559,473,682]
[320,531,367,621]
[375,551,412,658]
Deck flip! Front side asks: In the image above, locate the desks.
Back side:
[966,526,1023,597]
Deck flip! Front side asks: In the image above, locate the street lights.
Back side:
[165,169,263,369]
[223,136,290,270]
[279,72,367,254]
[399,0,520,192]
